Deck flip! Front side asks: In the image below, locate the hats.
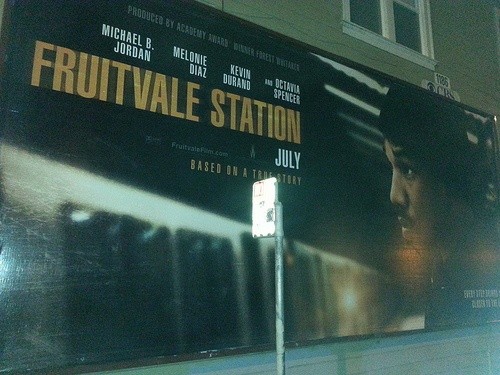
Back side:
[377,87,472,150]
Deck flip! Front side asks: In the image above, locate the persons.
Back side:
[376,82,500,332]
